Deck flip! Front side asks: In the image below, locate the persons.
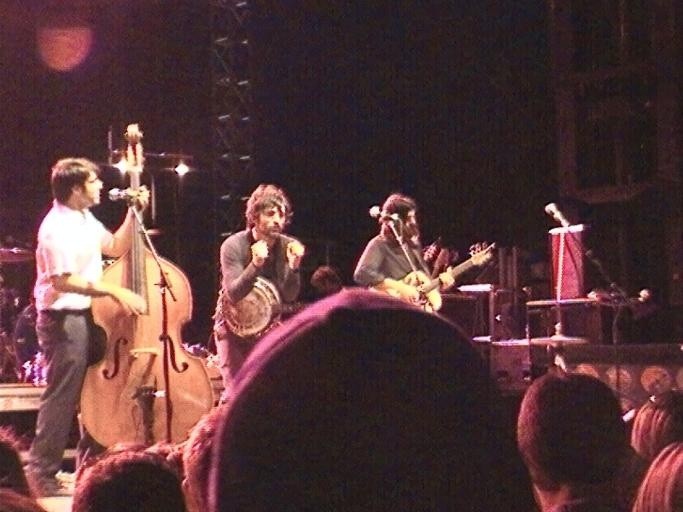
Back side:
[22,157,153,497]
[633,443,682,511]
[72,406,220,511]
[517,369,638,510]
[1,438,45,511]
[632,389,682,462]
[210,285,538,510]
[352,193,458,318]
[209,183,306,388]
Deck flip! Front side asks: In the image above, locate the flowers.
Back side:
[543,201,566,227]
[107,186,139,205]
[367,202,401,227]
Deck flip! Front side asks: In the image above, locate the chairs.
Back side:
[25,473,76,496]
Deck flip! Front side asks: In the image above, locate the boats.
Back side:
[437,287,683,410]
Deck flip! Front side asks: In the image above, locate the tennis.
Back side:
[80,123,215,447]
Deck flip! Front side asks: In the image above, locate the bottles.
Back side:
[208,287,540,512]
